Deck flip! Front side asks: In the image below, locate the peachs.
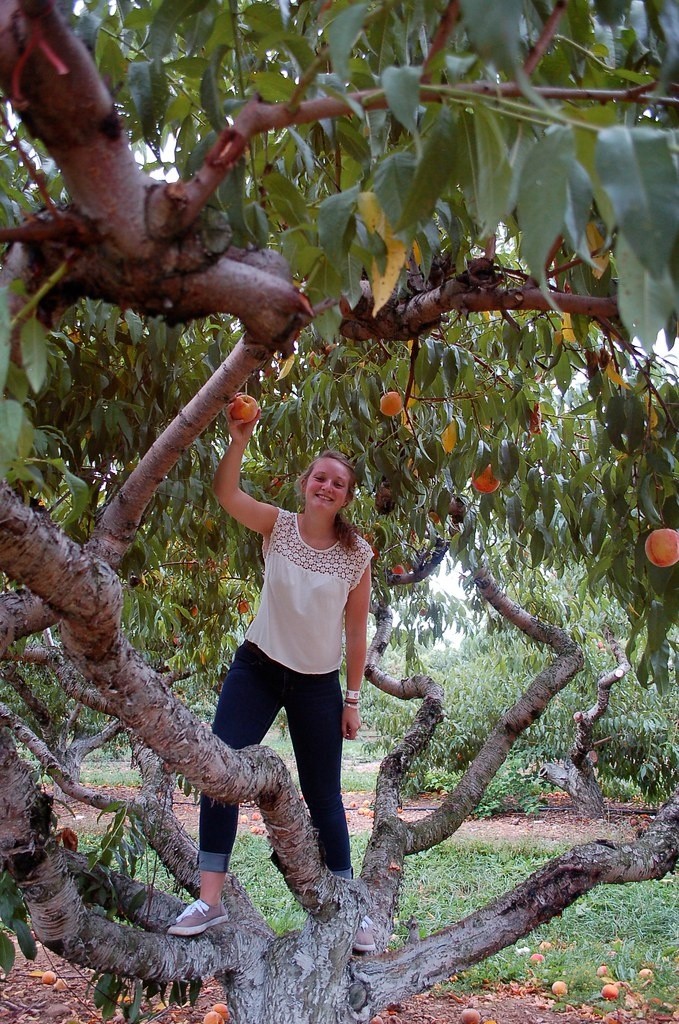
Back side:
[40,345,679,1024]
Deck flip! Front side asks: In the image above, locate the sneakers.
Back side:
[167,898,228,936]
[353,922,377,952]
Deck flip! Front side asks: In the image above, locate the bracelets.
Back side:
[343,689,359,710]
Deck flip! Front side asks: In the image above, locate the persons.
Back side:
[168,401,378,936]
